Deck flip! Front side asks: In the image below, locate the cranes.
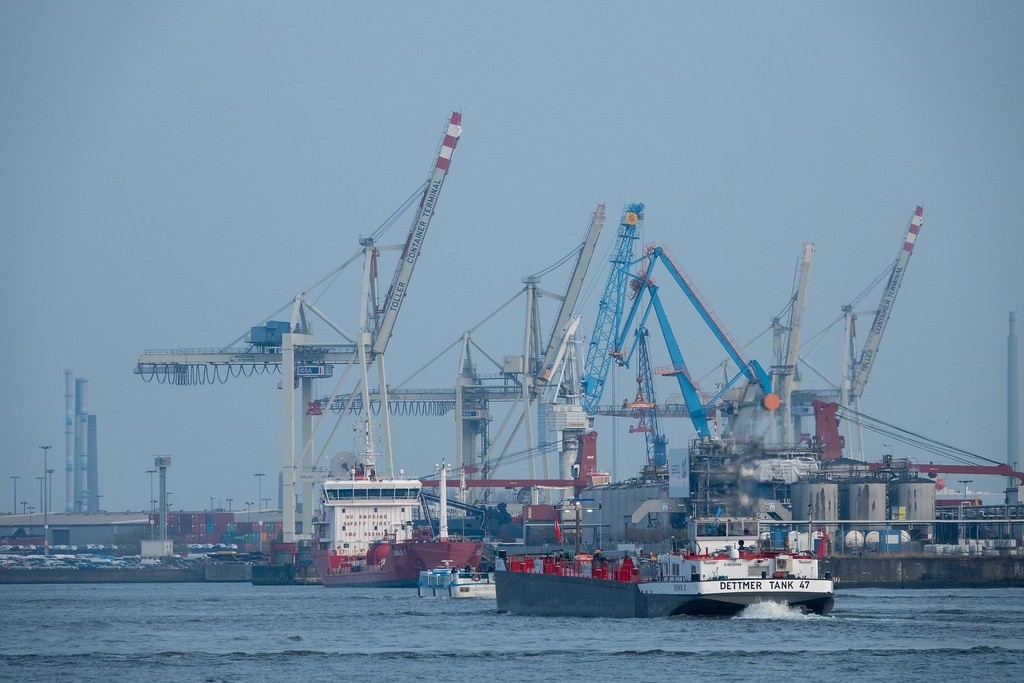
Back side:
[2,106,1022,598]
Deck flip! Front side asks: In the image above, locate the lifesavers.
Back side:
[473,573,481,581]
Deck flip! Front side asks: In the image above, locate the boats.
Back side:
[492,515,837,619]
